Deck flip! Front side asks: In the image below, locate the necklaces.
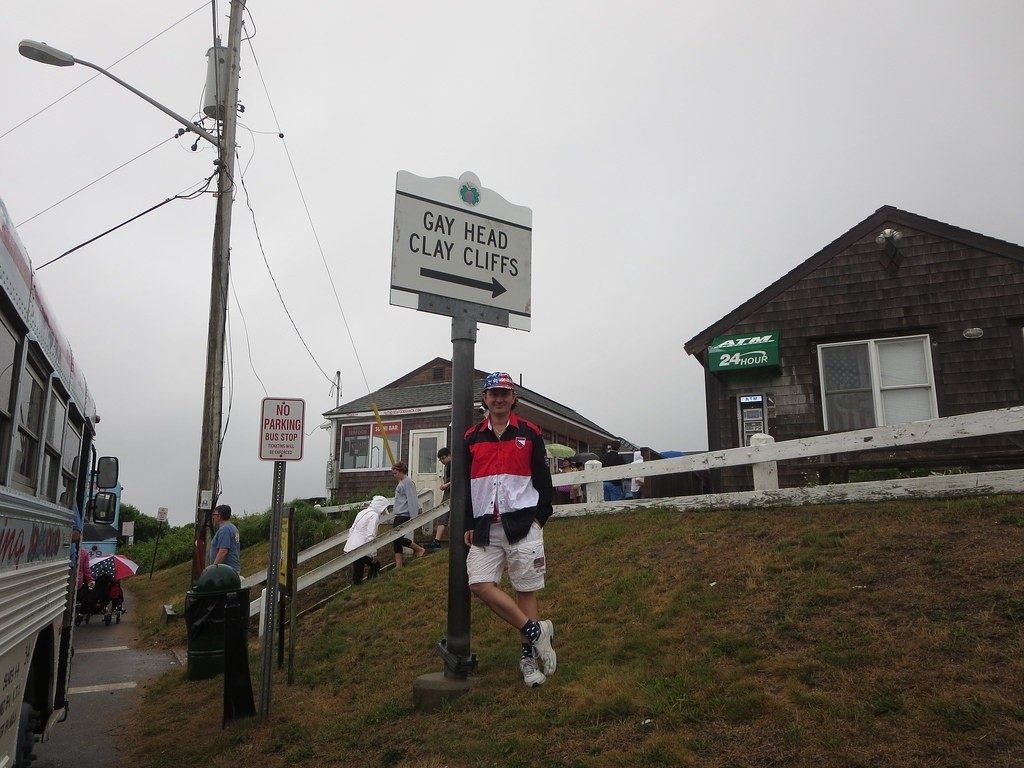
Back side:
[494,428,505,434]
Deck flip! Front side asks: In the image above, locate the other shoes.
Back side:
[424,545,442,552]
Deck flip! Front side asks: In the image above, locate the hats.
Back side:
[481,371,516,393]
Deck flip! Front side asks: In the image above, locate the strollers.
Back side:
[74,574,126,627]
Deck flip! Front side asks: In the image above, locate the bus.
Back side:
[82,469,124,562]
[0,192,121,768]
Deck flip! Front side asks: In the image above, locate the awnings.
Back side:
[708,331,782,373]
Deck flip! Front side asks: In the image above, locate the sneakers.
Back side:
[520,657,546,688]
[527,619,557,676]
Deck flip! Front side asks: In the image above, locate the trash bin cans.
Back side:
[183,563,252,682]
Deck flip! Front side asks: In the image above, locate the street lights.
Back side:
[17,38,244,592]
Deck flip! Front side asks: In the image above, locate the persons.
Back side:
[78,548,96,590]
[424,448,451,551]
[631,451,643,499]
[601,441,627,501]
[209,505,241,577]
[77,575,124,614]
[464,372,557,687]
[55,495,82,710]
[392,461,426,567]
[343,495,389,584]
[552,459,585,504]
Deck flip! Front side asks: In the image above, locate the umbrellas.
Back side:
[83,522,118,542]
[544,444,575,459]
[88,553,139,582]
[572,452,599,463]
[659,450,685,457]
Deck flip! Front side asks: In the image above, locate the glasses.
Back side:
[439,455,447,461]
[212,513,219,516]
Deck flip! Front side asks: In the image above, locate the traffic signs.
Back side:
[388,170,533,332]
